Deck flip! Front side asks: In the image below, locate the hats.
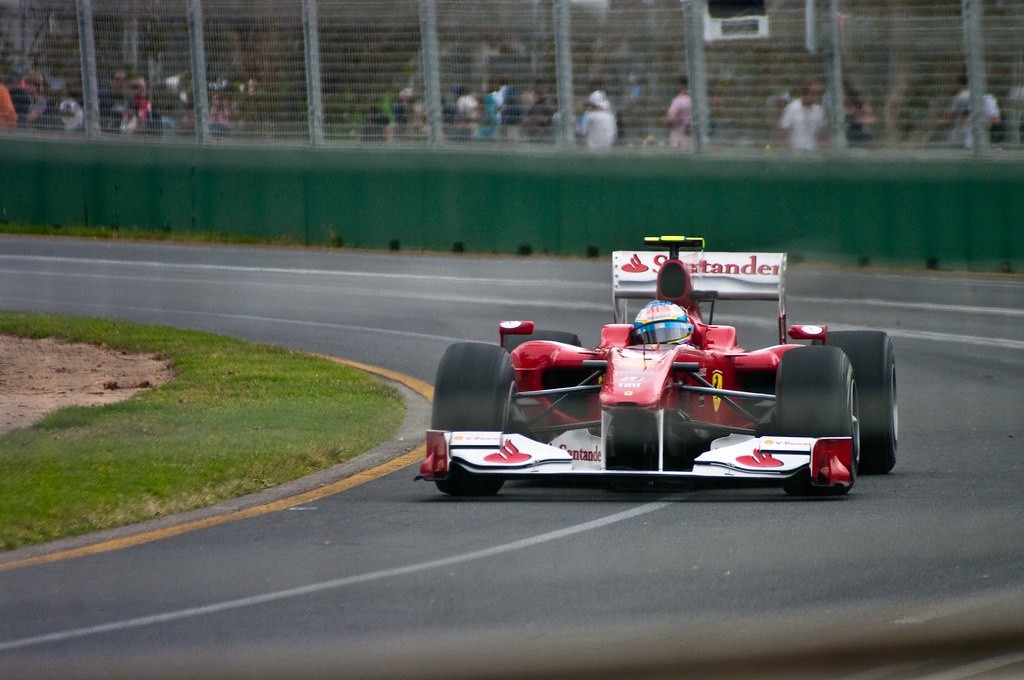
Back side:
[589,90,611,112]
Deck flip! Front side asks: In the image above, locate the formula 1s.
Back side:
[412,235,900,501]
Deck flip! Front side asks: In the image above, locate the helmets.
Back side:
[631,299,693,344]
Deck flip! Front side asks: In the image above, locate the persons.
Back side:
[594,299,706,367]
[0,46,1024,185]
